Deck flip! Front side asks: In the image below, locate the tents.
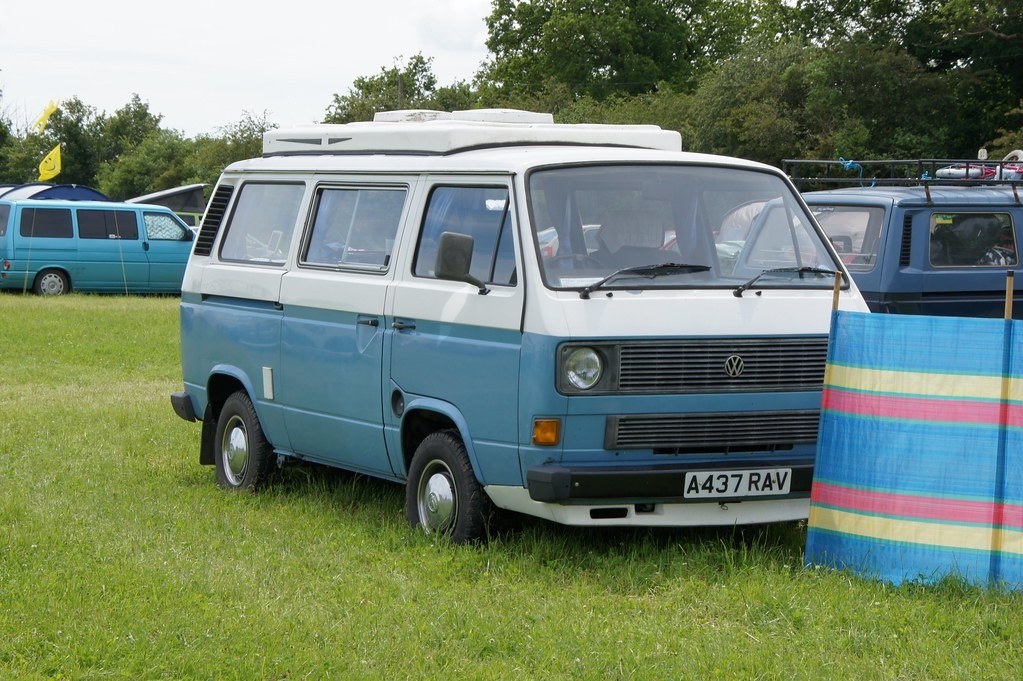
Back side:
[715,197,937,261]
[1,182,111,201]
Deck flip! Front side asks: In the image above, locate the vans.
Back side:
[170,106,882,545]
[0,198,199,297]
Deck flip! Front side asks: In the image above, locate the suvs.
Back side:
[730,147,1023,319]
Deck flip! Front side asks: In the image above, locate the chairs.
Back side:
[597,217,665,268]
